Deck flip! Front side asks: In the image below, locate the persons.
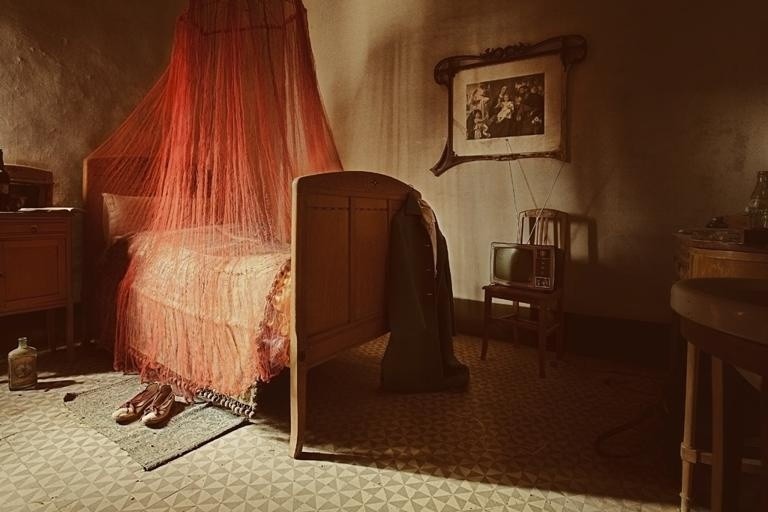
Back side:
[466,79,544,139]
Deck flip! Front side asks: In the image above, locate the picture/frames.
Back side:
[428,33,588,177]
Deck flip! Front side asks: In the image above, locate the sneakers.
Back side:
[115,383,160,424]
[142,383,175,426]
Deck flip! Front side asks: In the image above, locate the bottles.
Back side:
[747,172,768,230]
[7,337,38,391]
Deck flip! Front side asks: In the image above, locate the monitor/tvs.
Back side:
[489,241,558,292]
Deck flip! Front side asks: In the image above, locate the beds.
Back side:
[82,153,423,459]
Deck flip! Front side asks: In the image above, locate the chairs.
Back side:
[481,208,569,378]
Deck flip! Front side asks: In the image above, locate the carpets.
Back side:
[63,374,248,472]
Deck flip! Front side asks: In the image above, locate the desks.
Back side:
[668,275,768,512]
[0,209,77,367]
[671,229,768,511]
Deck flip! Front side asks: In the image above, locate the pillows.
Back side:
[101,192,220,238]
